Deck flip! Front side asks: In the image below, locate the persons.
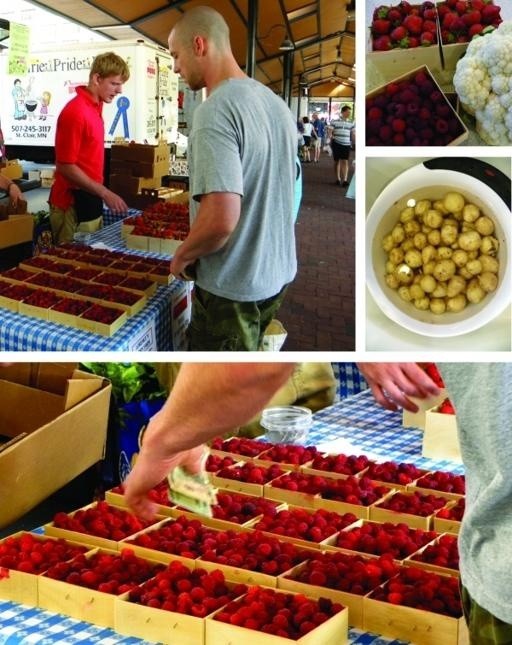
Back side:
[170,363,441,439]
[12,79,31,120]
[0,172,22,209]
[37,92,51,121]
[168,6,298,350]
[297,105,355,187]
[123,363,512,645]
[47,52,130,246]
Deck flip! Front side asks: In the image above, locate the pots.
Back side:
[25,100,37,111]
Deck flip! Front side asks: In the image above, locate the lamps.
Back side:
[257,24,294,50]
[295,72,307,85]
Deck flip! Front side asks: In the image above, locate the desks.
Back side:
[0,207,194,351]
[0,362,465,645]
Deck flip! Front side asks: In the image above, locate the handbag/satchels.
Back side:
[260,320,287,351]
[66,188,103,221]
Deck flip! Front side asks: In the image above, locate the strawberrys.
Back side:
[42,241,176,277]
[1,277,124,326]
[121,203,191,241]
[207,436,457,494]
[366,0,437,50]
[435,0,501,41]
[1,531,346,640]
[196,441,457,523]
[0,252,154,307]
[48,499,457,617]
[99,470,459,570]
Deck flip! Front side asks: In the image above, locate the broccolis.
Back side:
[453,22,512,146]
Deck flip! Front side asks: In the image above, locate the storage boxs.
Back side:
[0,215,32,249]
[0,361,112,525]
[1,159,57,188]
[110,137,183,208]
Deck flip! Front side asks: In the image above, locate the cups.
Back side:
[74,232,90,245]
[260,406,313,444]
[365,156,512,339]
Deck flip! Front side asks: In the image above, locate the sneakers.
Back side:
[337,180,350,187]
[301,159,319,163]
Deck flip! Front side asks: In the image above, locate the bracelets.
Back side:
[8,182,15,193]
[137,416,186,464]
